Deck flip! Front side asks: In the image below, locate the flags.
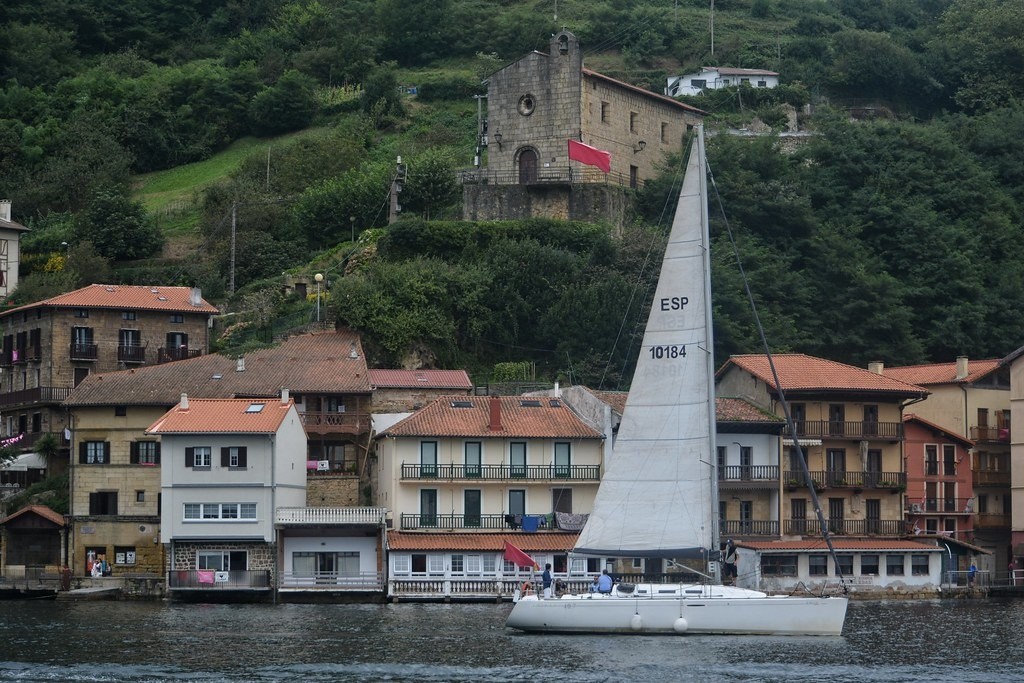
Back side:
[568,138,612,173]
[503,540,540,570]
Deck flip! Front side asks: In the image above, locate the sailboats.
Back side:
[504,118,854,637]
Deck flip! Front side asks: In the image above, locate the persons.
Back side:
[542,563,553,598]
[59,564,73,589]
[590,569,612,593]
[91,559,102,577]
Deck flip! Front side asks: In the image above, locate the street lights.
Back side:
[315,274,323,321]
